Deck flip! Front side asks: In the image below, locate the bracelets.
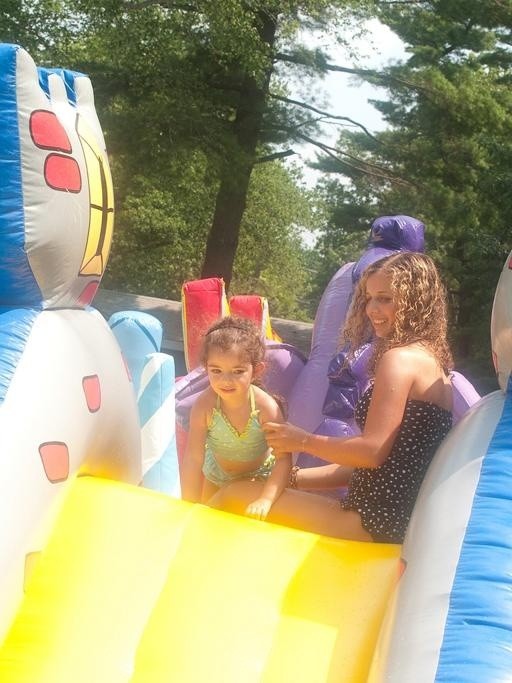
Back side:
[302,432,310,453]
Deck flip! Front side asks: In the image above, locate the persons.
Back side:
[179,320,291,522]
[265,253,453,543]
